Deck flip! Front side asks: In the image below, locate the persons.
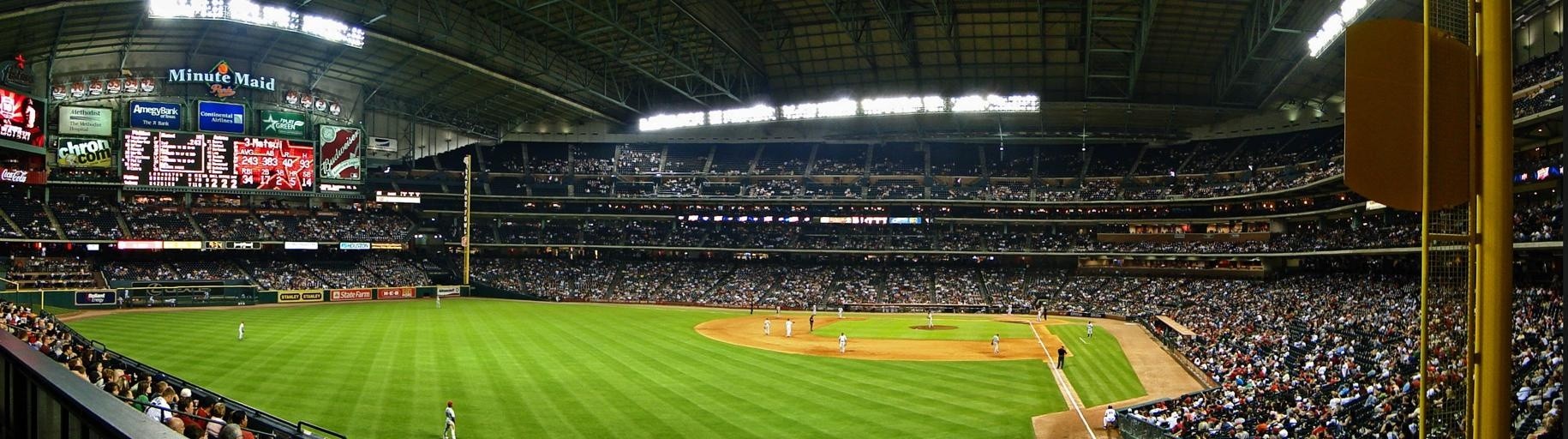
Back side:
[1102,404,1117,433]
[1121,332,1568,439]
[784,318,795,337]
[435,293,442,310]
[16,96,44,148]
[456,136,1566,333]
[1513,45,1565,121]
[763,318,771,337]
[1,165,457,294]
[990,333,1000,354]
[837,332,849,353]
[1085,320,1095,338]
[442,398,458,439]
[1055,344,1068,369]
[1,285,259,439]
